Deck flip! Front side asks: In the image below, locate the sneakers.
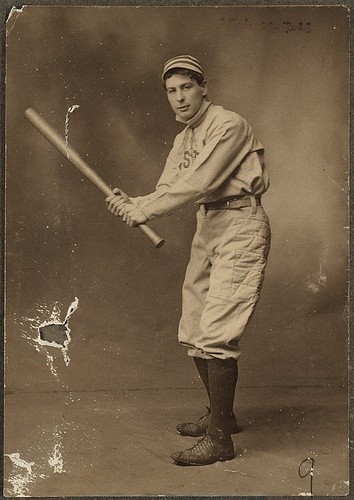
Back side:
[170,430,235,466]
[176,407,240,437]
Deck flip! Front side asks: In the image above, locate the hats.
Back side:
[161,54,204,83]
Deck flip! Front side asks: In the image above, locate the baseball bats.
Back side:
[24,107,165,248]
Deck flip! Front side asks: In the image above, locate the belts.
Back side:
[197,198,261,210]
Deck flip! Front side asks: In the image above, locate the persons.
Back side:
[103,54,273,468]
